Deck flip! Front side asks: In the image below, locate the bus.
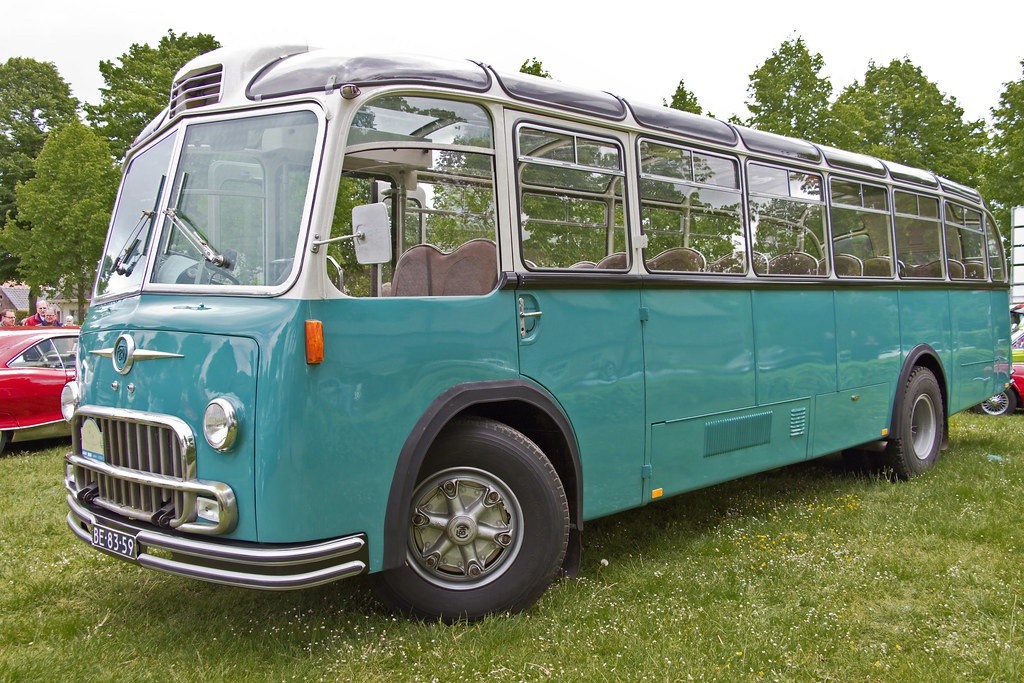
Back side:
[61,35,1018,617]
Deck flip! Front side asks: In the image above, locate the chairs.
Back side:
[768,252,820,275]
[962,262,993,280]
[524,259,536,268]
[819,253,863,276]
[593,251,627,269]
[47,354,76,362]
[569,261,597,270]
[643,247,707,273]
[863,256,906,277]
[921,258,965,278]
[711,251,770,274]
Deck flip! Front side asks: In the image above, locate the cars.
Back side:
[0,324,84,457]
[970,304,1024,417]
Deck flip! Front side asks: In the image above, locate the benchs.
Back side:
[390,238,498,296]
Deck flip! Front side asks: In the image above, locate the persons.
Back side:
[25,301,49,326]
[66,316,74,326]
[0,309,15,327]
[36,309,61,326]
